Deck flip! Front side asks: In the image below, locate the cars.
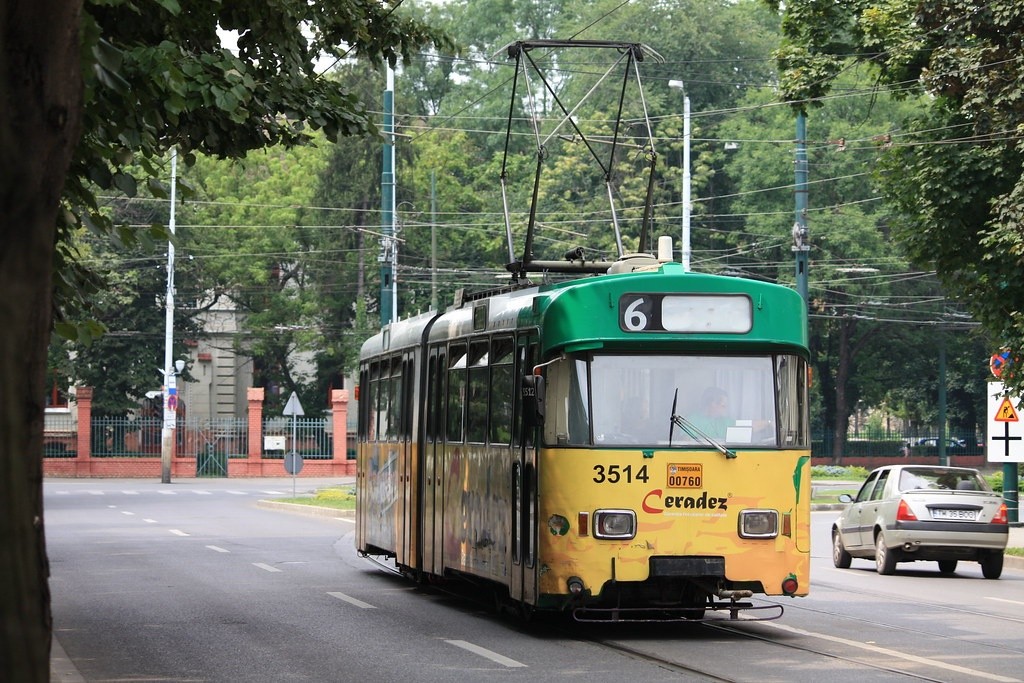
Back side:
[832,465,1008,579]
[952,439,983,447]
[915,438,963,448]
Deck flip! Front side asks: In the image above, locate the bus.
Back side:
[353,263,811,625]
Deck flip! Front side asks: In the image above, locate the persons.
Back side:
[679,387,736,441]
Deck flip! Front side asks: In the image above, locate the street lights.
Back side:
[667,79,690,272]
[160,359,184,483]
